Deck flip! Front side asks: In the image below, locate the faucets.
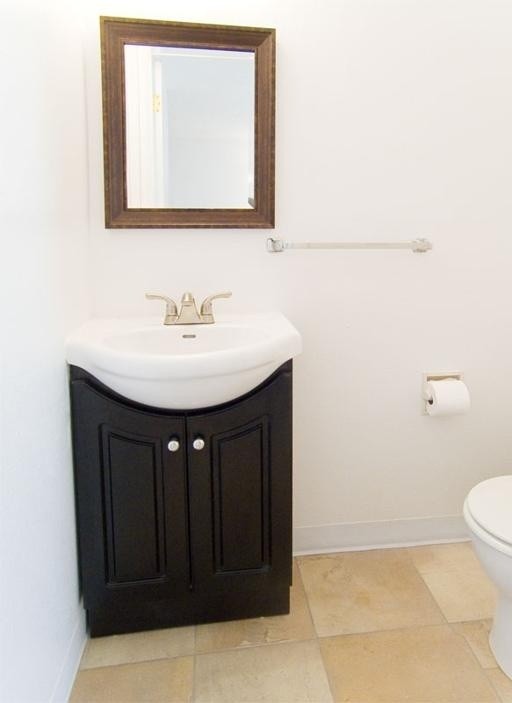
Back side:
[178,292,201,325]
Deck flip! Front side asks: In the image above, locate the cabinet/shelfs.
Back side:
[69,372,294,639]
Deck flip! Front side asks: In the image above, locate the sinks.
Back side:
[68,312,303,411]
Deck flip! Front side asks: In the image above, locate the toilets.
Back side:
[461,476,512,681]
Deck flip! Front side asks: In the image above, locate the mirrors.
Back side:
[99,18,275,228]
